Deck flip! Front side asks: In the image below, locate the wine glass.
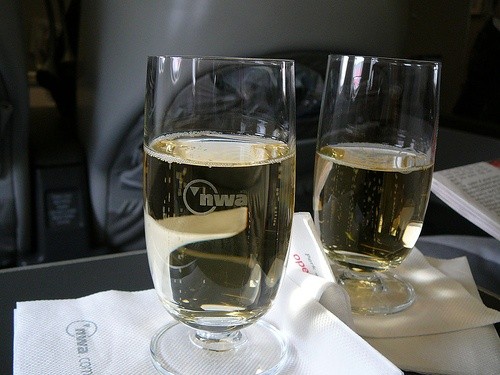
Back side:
[140,54,300,374]
[308,52,443,320]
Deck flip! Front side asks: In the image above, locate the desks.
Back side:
[0,235,500,375]
